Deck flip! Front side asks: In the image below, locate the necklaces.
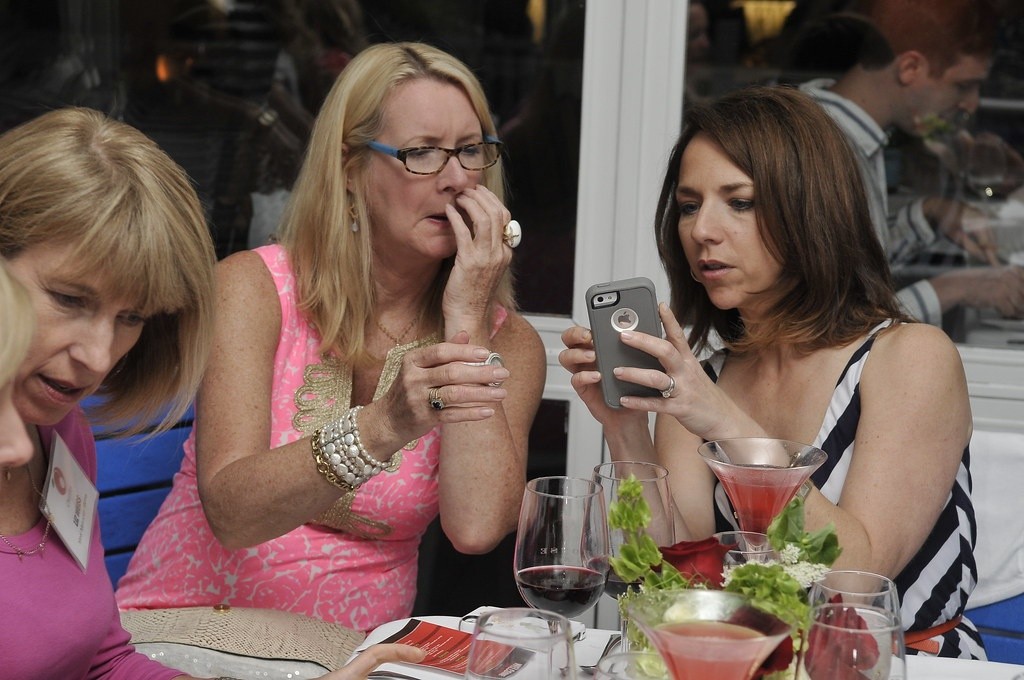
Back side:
[373,311,421,346]
[0,463,55,559]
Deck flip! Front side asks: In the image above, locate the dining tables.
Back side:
[345,605,1024,680]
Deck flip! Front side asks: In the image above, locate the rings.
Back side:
[659,375,675,398]
[428,388,445,409]
[503,220,521,248]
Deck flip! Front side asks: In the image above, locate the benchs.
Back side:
[957,430,1024,666]
[82,384,196,592]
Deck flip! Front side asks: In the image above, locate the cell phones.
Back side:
[585,277,667,409]
[458,606,586,654]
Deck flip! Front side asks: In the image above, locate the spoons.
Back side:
[579,634,623,675]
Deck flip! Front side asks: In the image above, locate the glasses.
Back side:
[364,136,504,177]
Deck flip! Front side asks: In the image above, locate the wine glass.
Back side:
[967,138,1006,215]
[697,436,828,562]
[579,460,677,680]
[513,476,610,680]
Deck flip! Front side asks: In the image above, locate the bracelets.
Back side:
[310,405,392,492]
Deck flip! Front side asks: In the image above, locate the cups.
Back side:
[712,530,776,565]
[465,608,577,680]
[795,570,907,680]
[592,652,674,680]
[626,589,792,680]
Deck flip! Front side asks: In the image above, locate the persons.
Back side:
[145,0,536,257]
[687,0,1024,328]
[113,42,547,632]
[0,256,34,467]
[0,108,424,680]
[558,88,988,663]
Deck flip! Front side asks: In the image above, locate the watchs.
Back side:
[465,352,505,387]
